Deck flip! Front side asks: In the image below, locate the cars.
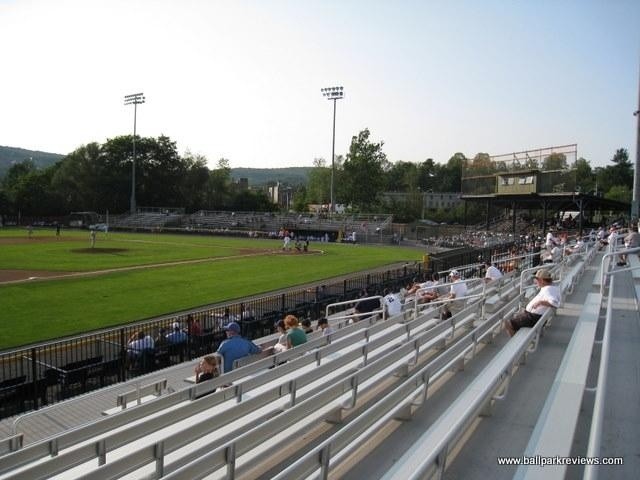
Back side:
[0,221,60,228]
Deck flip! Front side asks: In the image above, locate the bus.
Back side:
[68,211,99,228]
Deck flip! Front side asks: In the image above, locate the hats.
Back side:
[223,322,241,333]
[531,269,552,279]
[173,322,179,329]
[449,270,460,277]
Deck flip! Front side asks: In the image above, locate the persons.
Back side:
[194,355,219,400]
[215,321,262,374]
[126,285,374,359]
[374,220,639,336]
[278,227,357,254]
[90,228,97,248]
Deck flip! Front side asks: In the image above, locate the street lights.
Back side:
[417,186,433,220]
[320,86,344,215]
[123,93,146,216]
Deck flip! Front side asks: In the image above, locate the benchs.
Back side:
[0,230,639,480]
